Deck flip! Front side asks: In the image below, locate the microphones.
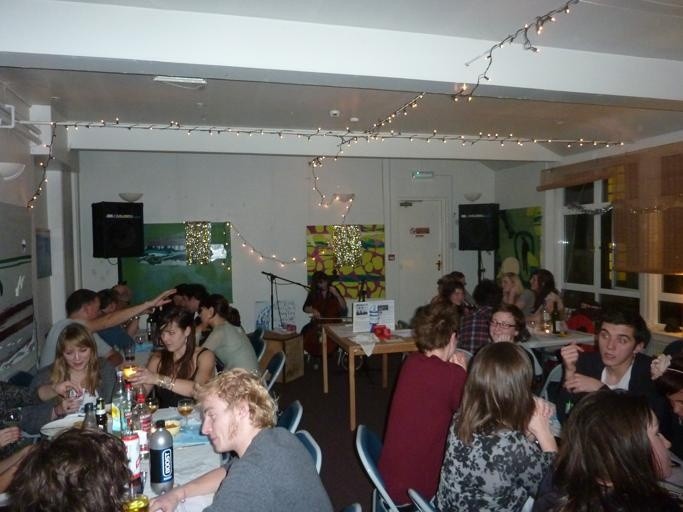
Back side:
[316,284,320,292]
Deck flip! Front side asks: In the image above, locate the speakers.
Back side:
[91,201,144,258]
[458,203,500,251]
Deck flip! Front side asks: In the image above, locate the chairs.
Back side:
[222,328,323,482]
[345,423,441,512]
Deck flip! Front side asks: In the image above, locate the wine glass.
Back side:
[136,336,145,351]
[528,320,536,337]
[177,399,194,431]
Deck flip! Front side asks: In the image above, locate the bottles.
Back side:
[540,306,551,336]
[146,313,153,340]
[562,397,573,421]
[81,370,174,496]
[552,302,560,334]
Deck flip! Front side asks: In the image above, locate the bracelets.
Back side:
[177,482,187,503]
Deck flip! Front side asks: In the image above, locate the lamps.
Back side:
[1,162,26,182]
[118,191,143,203]
[333,193,355,204]
[464,192,483,201]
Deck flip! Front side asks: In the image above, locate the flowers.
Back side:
[650,354,682,380]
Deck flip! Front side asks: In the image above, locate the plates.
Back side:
[40,418,83,438]
[394,329,411,338]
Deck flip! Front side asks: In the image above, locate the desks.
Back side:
[516,326,598,398]
[321,323,418,431]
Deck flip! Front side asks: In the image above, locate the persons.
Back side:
[377,305,470,511]
[1,282,266,491]
[413,266,682,475]
[534,386,683,512]
[146,368,334,512]
[300,272,349,369]
[434,343,558,512]
[5,429,130,512]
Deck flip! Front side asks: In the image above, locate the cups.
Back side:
[121,495,148,512]
[124,363,140,382]
[3,410,22,427]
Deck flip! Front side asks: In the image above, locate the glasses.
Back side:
[490,321,517,328]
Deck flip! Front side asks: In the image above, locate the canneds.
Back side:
[121,434,140,481]
